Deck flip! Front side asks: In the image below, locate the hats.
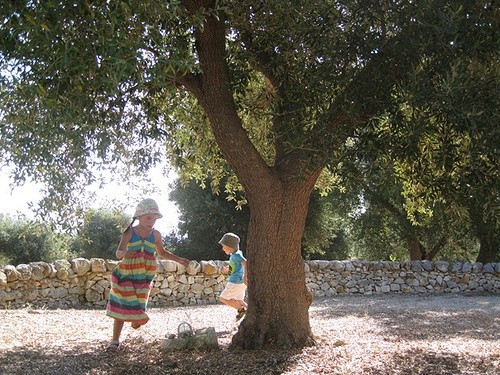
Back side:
[132,198,163,220]
[218,233,240,251]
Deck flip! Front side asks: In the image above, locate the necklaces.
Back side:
[134,227,154,252]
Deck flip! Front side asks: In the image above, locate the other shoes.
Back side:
[234,306,247,322]
[107,338,120,351]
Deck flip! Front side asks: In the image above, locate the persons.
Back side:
[106,198,189,350]
[218,232,248,322]
